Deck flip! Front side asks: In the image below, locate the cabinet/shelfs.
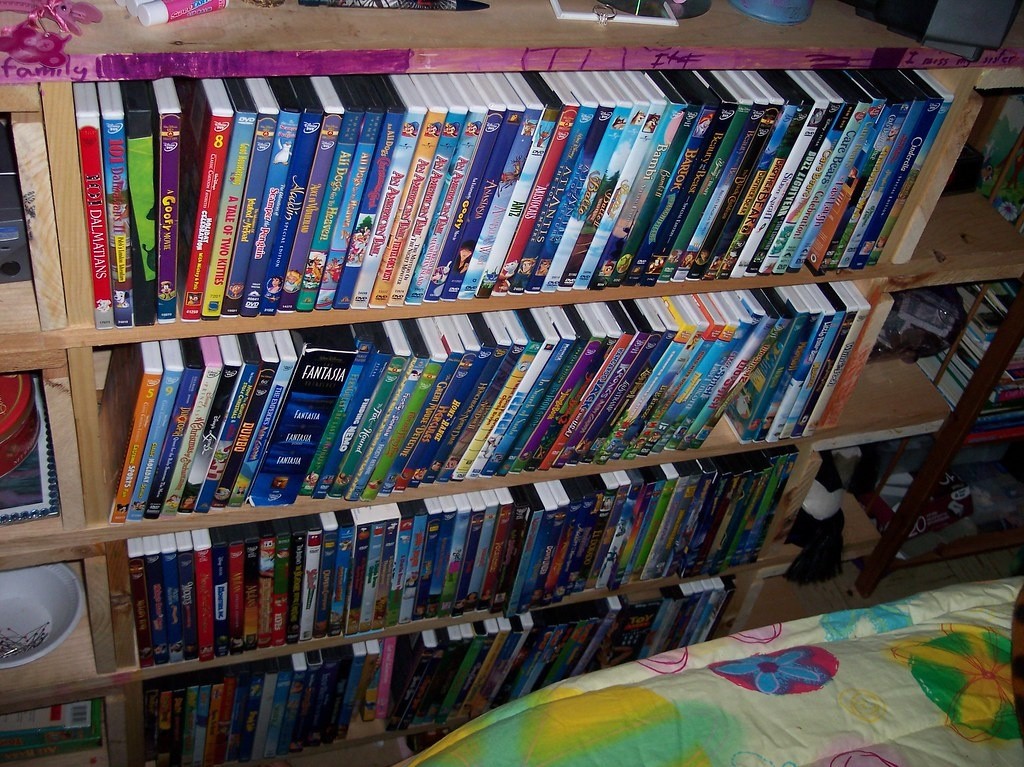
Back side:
[0,0,1023,767]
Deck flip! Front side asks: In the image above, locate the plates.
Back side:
[1,562,83,671]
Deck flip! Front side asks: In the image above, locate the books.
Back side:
[0,64,1024,767]
